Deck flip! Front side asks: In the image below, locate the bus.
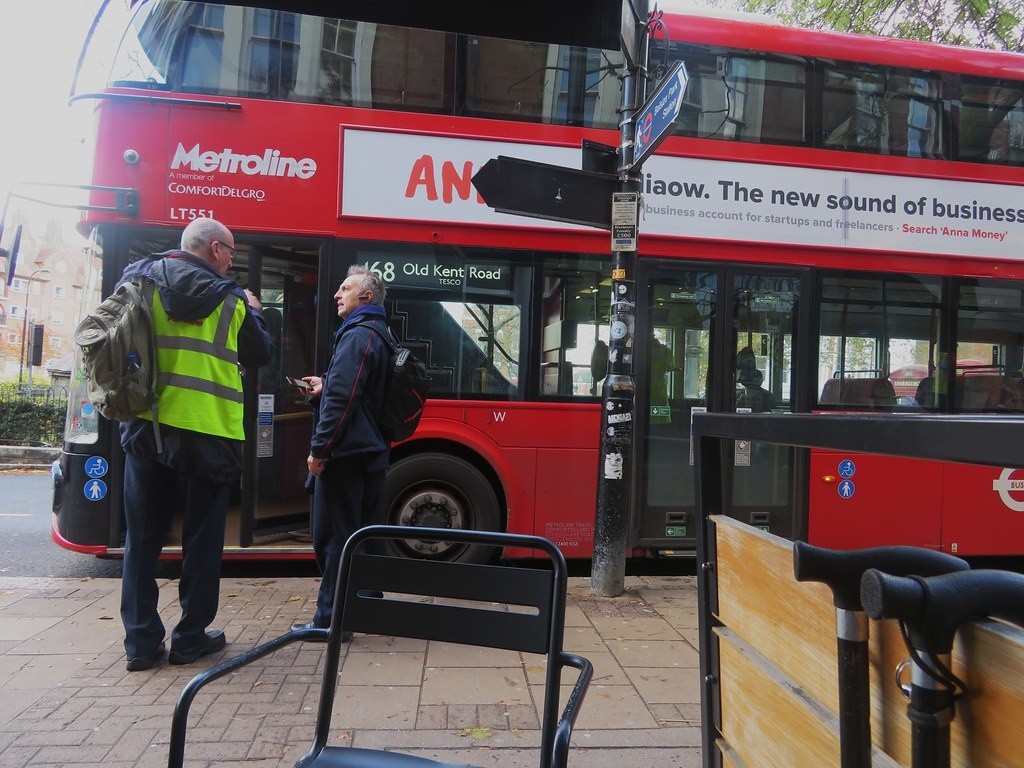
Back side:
[0,0,1024,564]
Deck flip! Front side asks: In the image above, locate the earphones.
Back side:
[358,296,368,300]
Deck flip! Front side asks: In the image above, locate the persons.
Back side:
[112,217,275,671]
[290,265,395,642]
[646,328,675,508]
[736,369,780,466]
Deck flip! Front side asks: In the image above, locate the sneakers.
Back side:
[167,630,226,666]
[126,640,165,672]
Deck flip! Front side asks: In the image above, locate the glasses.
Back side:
[208,240,238,260]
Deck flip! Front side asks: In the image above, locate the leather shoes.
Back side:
[289,621,353,642]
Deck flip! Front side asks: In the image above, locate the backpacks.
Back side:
[332,320,434,444]
[73,275,164,454]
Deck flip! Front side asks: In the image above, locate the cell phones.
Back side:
[286,376,314,391]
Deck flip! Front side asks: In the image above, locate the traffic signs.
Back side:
[633,60,690,173]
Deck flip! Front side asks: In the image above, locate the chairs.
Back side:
[167,524,593,768]
[822,363,1024,409]
